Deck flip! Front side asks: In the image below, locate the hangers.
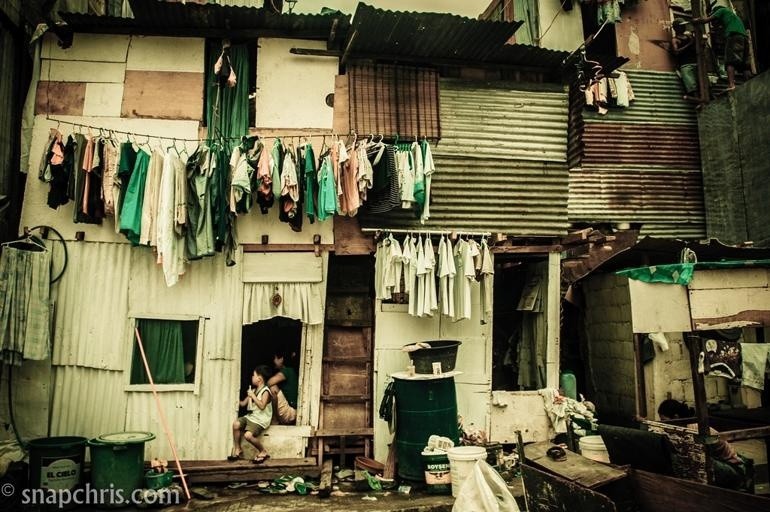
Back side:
[2,230,52,259]
[377,228,490,258]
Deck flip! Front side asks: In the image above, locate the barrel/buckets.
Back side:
[420,450,451,496]
[22,437,87,511]
[577,436,611,465]
[445,444,488,498]
[87,430,156,507]
[390,371,461,491]
[146,467,173,492]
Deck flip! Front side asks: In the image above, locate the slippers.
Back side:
[252,455,270,464]
[227,455,246,461]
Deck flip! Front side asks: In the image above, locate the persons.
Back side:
[693,5,750,92]
[657,399,746,492]
[226,364,275,464]
[670,17,707,101]
[266,345,298,427]
